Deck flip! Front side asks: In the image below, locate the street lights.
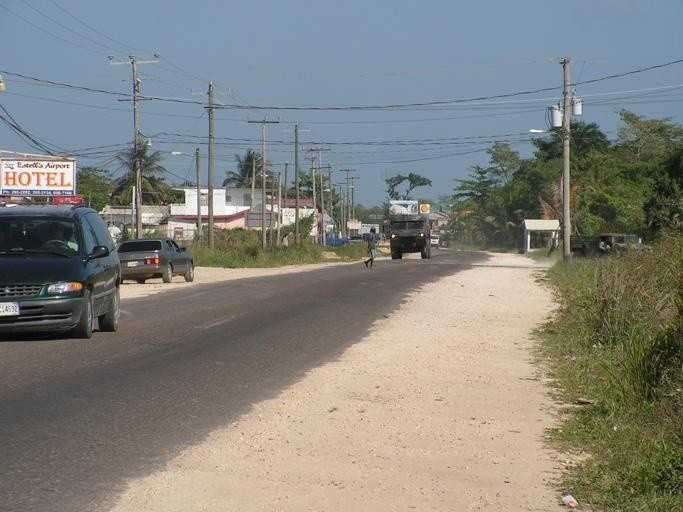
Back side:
[170,150,196,158]
[528,127,564,141]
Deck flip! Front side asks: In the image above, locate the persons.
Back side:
[362,226,378,270]
[281,233,288,248]
[44,224,79,253]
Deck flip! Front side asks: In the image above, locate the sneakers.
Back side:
[364,261,372,268]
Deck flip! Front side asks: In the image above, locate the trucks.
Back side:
[568,232,652,259]
[388,213,440,260]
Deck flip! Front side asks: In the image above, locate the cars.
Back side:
[115,237,195,284]
[0,202,122,340]
